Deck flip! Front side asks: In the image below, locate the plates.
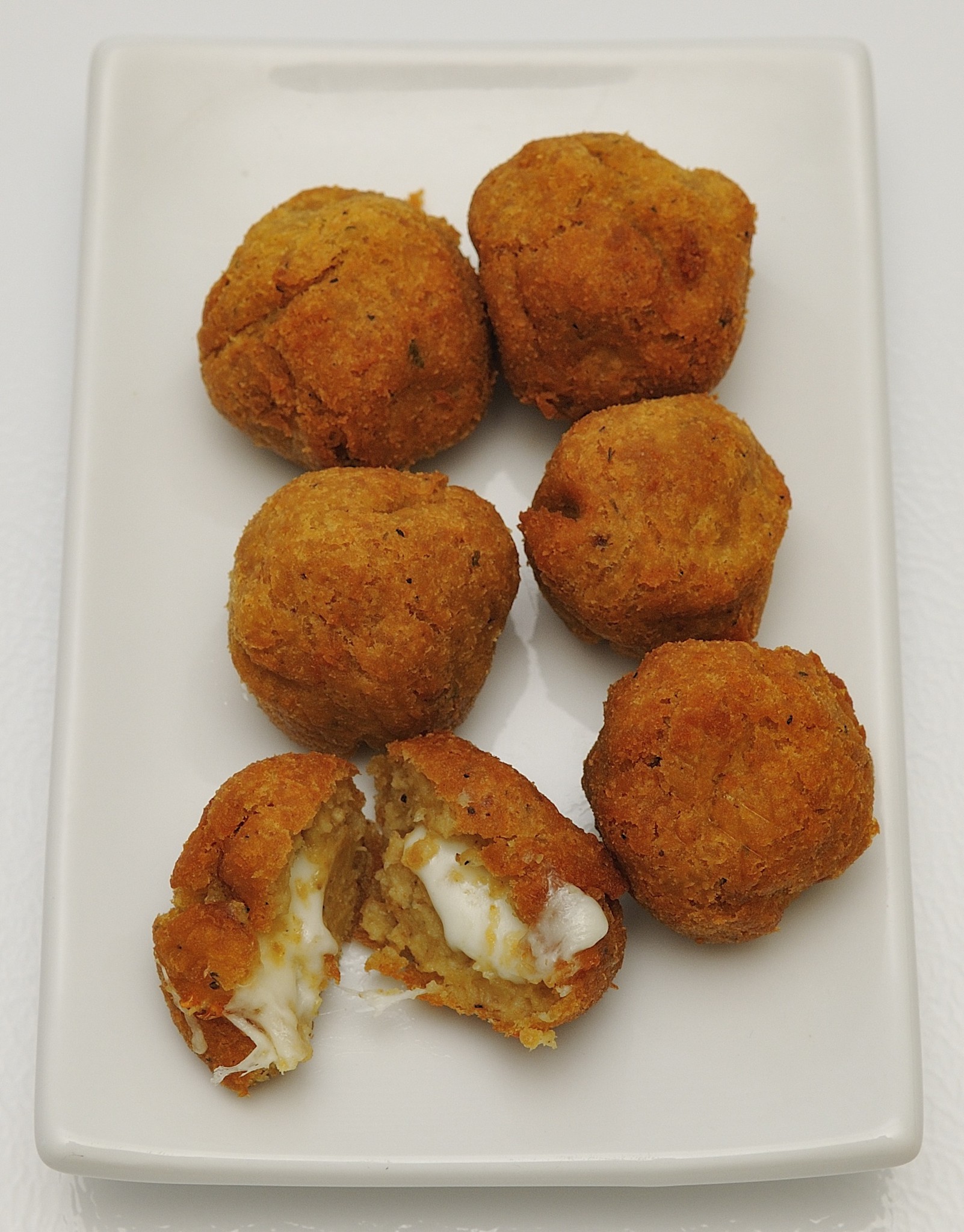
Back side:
[37,41,924,1187]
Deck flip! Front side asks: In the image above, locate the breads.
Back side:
[517,393,791,660]
[198,186,498,469]
[227,467,521,754]
[467,131,759,420]
[151,731,627,1098]
[583,639,879,944]
